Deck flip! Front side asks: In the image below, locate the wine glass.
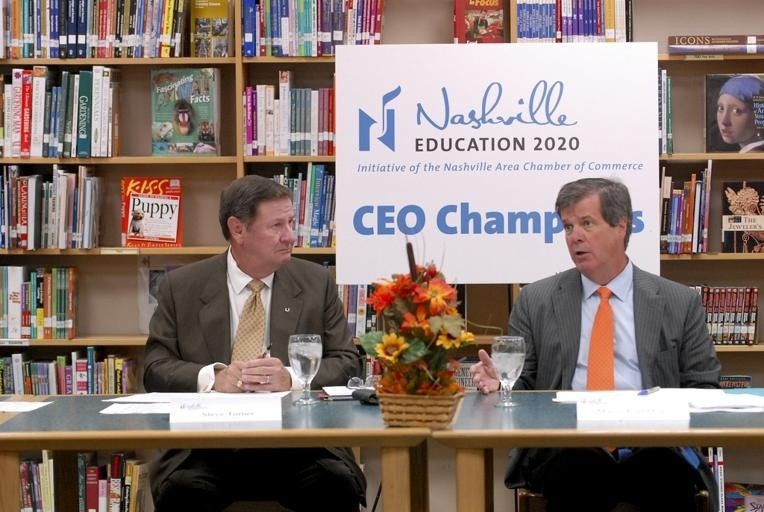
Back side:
[287,332,324,406]
[491,335,527,409]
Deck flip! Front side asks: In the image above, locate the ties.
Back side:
[585,287,618,452]
[230,280,265,362]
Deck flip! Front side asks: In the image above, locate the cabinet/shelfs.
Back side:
[0,2,764,511]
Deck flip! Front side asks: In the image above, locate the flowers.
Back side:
[359,261,476,396]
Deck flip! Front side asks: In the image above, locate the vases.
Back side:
[376,392,465,429]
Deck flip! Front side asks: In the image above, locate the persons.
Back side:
[714,74,764,154]
[469,177,723,512]
[143,175,364,511]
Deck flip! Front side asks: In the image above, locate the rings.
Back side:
[236,379,243,389]
[266,375,270,384]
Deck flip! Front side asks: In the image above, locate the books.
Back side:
[516,0,627,42]
[704,72,763,155]
[659,159,712,255]
[1,347,138,393]
[714,447,726,512]
[0,266,79,339]
[703,444,715,472]
[1,66,223,157]
[669,45,764,55]
[16,447,149,511]
[1,165,182,251]
[720,181,764,253]
[140,266,184,334]
[658,66,673,155]
[453,1,510,42]
[668,33,764,45]
[2,1,234,58]
[688,284,758,348]
[242,0,383,377]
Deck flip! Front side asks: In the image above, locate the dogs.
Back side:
[128,209,144,236]
